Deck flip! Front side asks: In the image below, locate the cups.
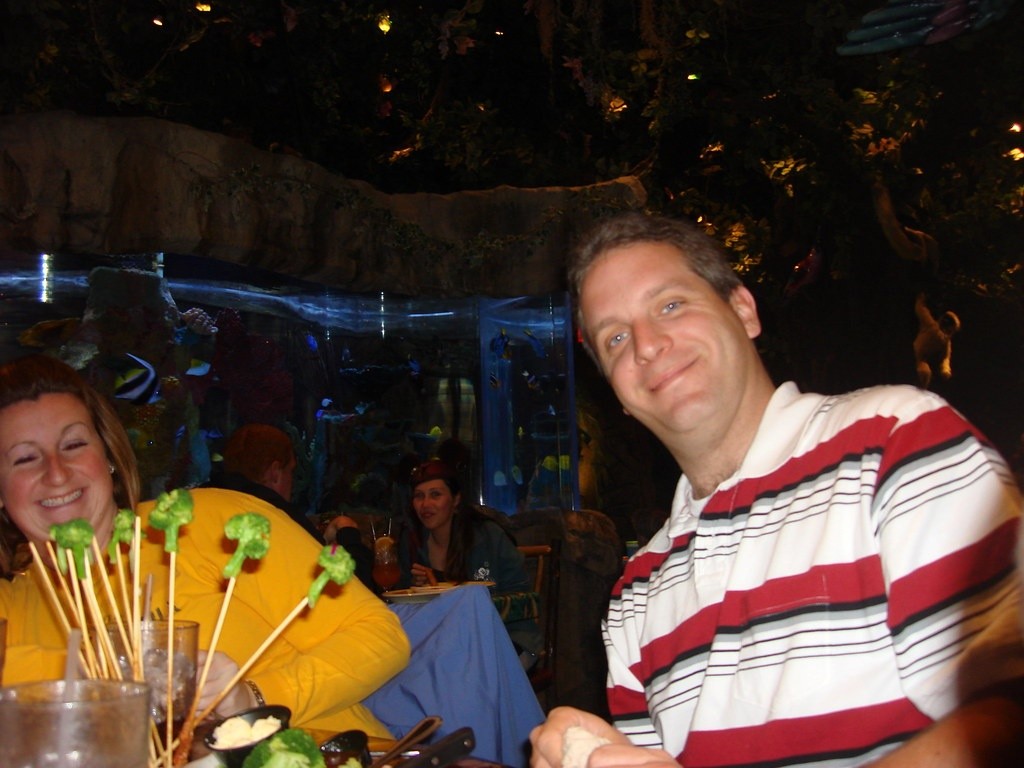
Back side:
[0,680,149,768]
[372,549,400,593]
[99,619,200,759]
[320,730,374,768]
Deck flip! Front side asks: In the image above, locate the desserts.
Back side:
[212,716,280,749]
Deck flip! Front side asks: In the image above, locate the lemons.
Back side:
[375,537,394,551]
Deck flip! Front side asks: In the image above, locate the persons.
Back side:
[781,135,1024,394]
[187,422,378,599]
[0,353,413,739]
[397,456,540,670]
[530,212,1024,767]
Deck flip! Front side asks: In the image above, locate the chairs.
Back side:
[516,544,554,696]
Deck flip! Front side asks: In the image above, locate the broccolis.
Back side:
[50,520,93,578]
[108,509,146,562]
[222,513,270,579]
[242,728,325,768]
[307,545,355,608]
[148,486,193,551]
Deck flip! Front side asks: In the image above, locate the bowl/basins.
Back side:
[204,705,292,768]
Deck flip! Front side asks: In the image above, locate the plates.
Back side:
[384,585,453,603]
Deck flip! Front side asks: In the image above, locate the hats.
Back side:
[409,460,447,488]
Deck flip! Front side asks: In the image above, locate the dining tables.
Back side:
[492,592,557,621]
[171,722,506,767]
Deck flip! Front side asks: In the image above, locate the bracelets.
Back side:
[244,679,265,707]
